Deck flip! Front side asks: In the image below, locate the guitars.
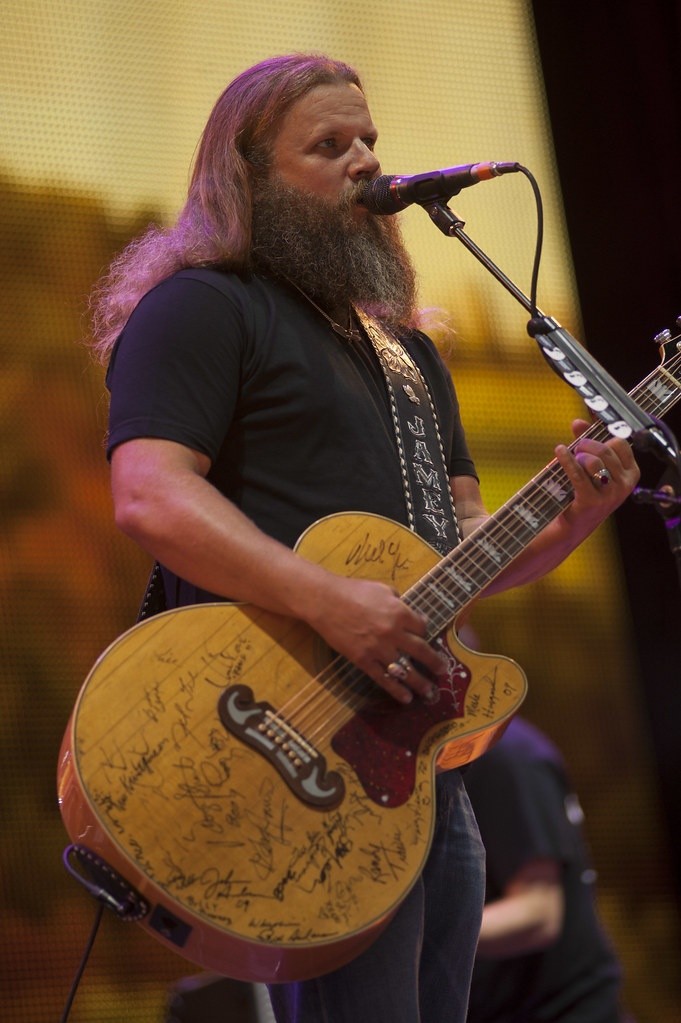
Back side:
[56,311,681,984]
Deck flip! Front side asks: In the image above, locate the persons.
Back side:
[460,712,624,1023]
[96,52,640,1023]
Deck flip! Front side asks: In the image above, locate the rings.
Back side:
[592,469,612,490]
[385,657,411,685]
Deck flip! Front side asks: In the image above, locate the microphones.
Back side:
[362,162,517,215]
